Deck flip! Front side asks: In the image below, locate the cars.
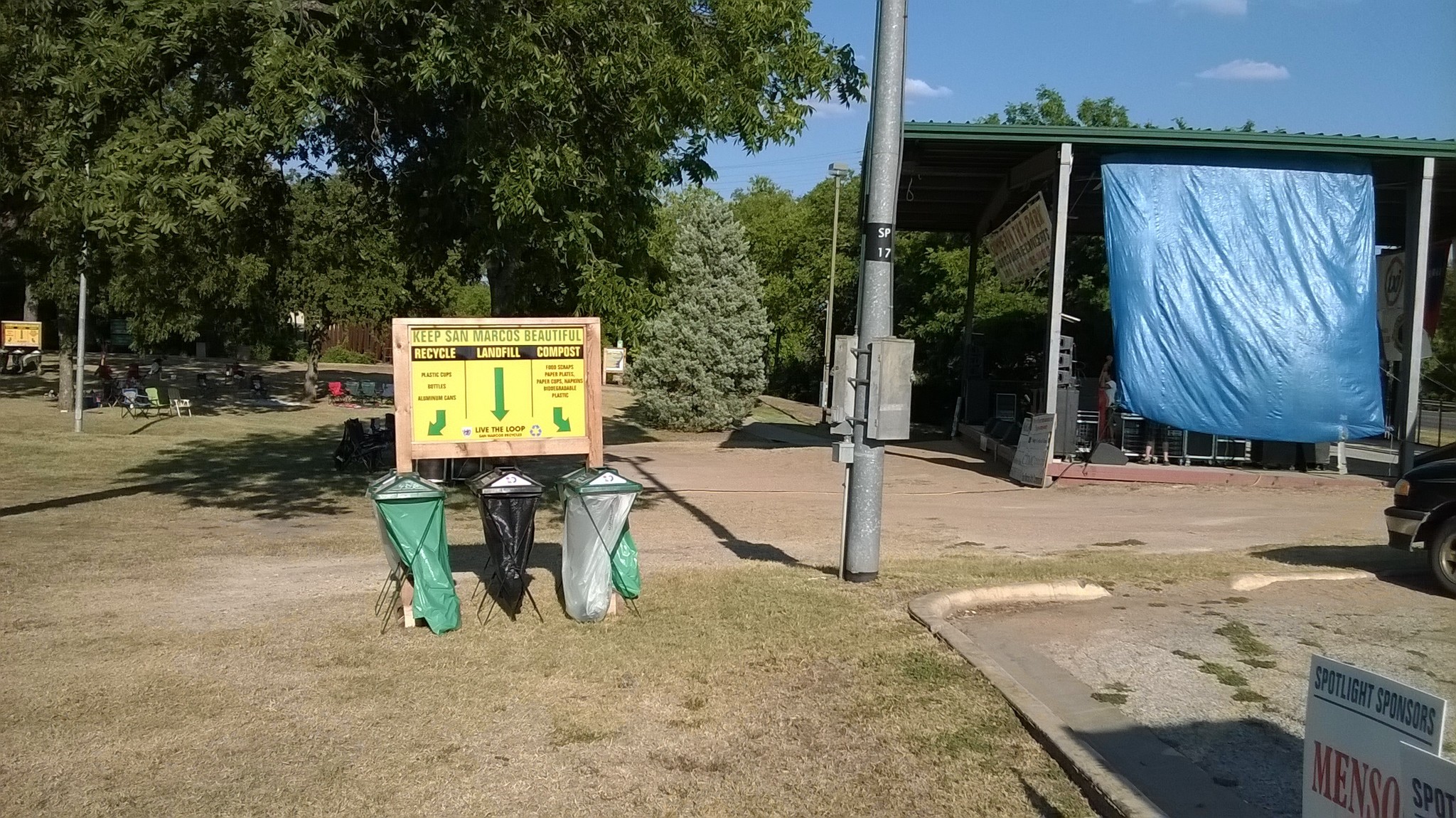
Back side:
[1384,458,1456,599]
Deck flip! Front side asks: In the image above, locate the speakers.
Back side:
[1052,389,1079,455]
[1056,370,1070,387]
[963,379,987,423]
[1059,335,1073,350]
[1089,440,1129,465]
[1255,441,1297,469]
[1059,352,1071,367]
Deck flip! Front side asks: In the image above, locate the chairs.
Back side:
[331,413,397,475]
[195,373,218,400]
[94,358,192,419]
[328,381,394,408]
[224,362,270,399]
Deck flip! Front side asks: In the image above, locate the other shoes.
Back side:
[1138,459,1149,464]
[1164,461,1171,466]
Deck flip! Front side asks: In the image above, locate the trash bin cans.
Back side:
[368,469,463,636]
[556,466,643,622]
[464,466,546,613]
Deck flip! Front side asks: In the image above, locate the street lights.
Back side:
[815,162,850,437]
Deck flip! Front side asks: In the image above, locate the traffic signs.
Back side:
[408,324,586,442]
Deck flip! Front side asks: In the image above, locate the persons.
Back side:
[1137,417,1170,465]
